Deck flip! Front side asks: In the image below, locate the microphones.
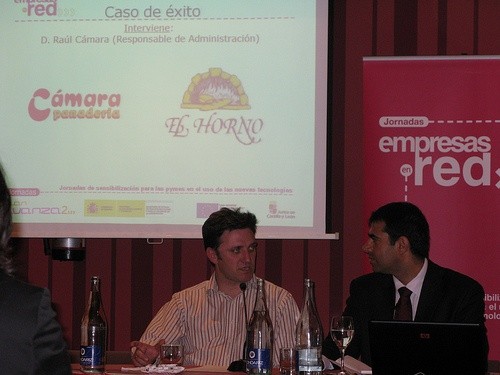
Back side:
[227,283,251,371]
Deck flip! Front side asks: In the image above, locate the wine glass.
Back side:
[330,316,355,375]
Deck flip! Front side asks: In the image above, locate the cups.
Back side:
[160,345,184,365]
[279,347,298,375]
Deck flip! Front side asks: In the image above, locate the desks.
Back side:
[68,361,359,375]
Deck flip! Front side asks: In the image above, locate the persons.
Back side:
[128,207,302,370]
[0,164,72,375]
[319,201,490,375]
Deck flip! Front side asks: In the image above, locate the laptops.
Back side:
[371,321,481,375]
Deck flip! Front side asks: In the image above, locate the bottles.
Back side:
[293,278,323,375]
[246,278,274,375]
[79,275,107,373]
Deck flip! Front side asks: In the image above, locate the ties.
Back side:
[395,287,412,322]
